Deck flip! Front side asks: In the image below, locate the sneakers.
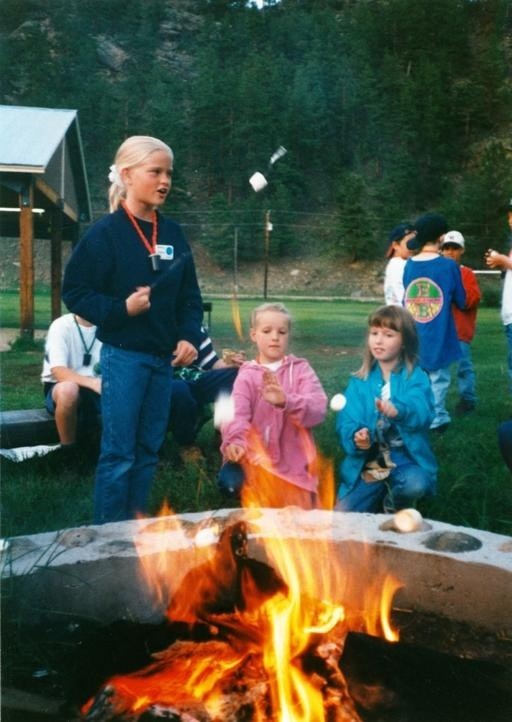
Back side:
[454,400,475,412]
[433,423,448,434]
[168,438,204,472]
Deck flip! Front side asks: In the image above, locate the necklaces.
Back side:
[72,315,97,366]
[118,198,162,272]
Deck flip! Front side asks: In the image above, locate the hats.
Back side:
[407,215,448,250]
[384,224,416,258]
[440,231,465,249]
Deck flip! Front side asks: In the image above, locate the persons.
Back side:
[60,134,205,526]
[169,318,247,465]
[384,210,482,432]
[220,299,328,508]
[333,305,442,514]
[484,199,512,392]
[39,314,103,474]
